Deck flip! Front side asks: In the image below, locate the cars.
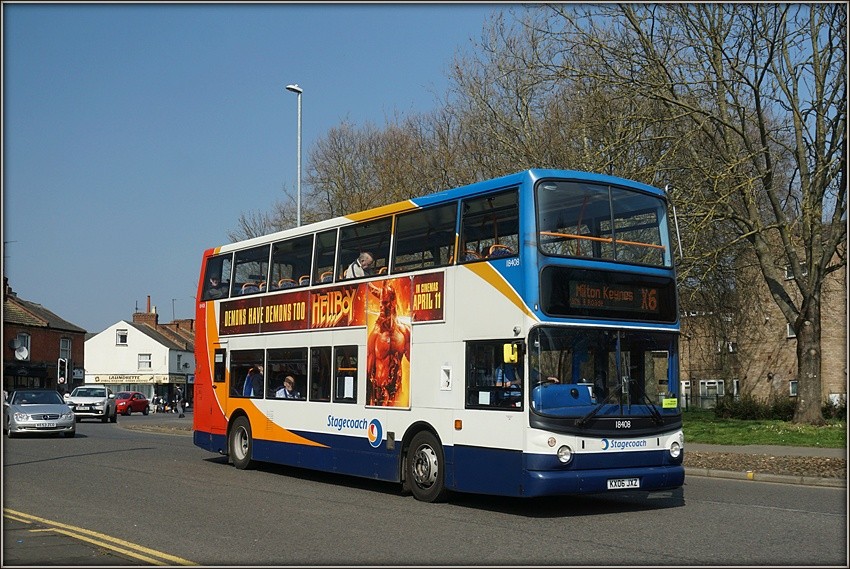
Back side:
[3,389,77,438]
[115,391,149,416]
[64,384,117,423]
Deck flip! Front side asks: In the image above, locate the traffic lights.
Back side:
[58,358,65,384]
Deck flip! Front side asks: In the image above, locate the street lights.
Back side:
[285,83,304,227]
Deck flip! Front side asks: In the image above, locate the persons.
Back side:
[346,250,374,278]
[367,279,410,406]
[152,394,159,414]
[249,365,263,396]
[495,349,545,398]
[276,376,301,399]
[177,388,185,418]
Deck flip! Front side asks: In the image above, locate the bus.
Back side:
[193,166,686,505]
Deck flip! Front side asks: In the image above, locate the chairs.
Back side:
[238,266,387,296]
[447,244,515,264]
